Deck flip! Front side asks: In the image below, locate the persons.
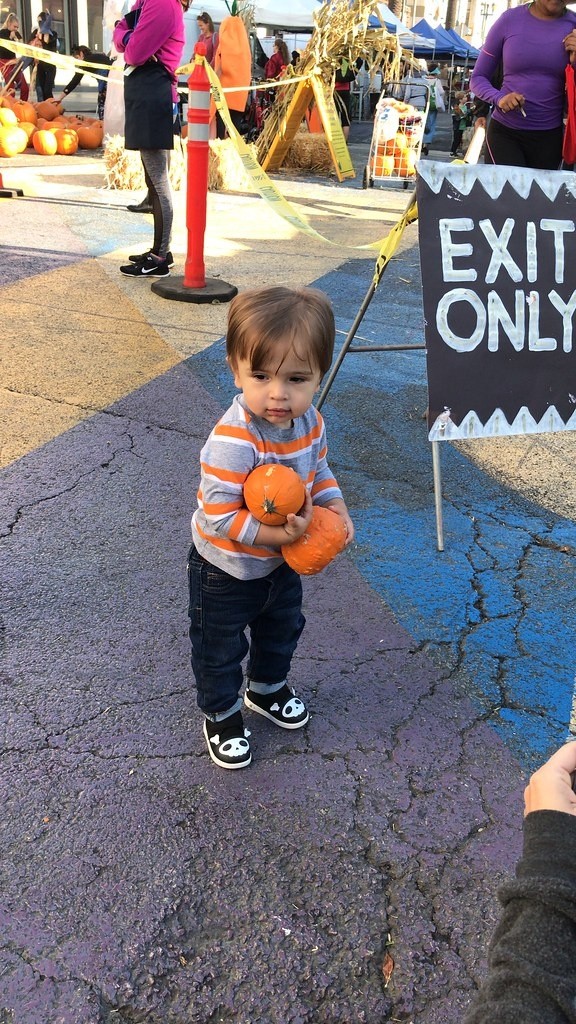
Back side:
[264,39,290,106]
[192,11,220,141]
[469,0,576,172]
[187,285,355,770]
[291,50,301,68]
[30,12,60,103]
[388,59,447,156]
[449,90,472,158]
[0,12,29,105]
[465,66,471,84]
[50,45,112,120]
[452,66,457,79]
[469,93,491,130]
[332,45,364,143]
[439,63,449,105]
[460,740,576,1024]
[112,0,193,278]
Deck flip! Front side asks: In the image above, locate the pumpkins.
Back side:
[0,88,103,158]
[370,100,420,177]
[243,463,305,526]
[281,505,349,575]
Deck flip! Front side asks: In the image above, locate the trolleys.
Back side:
[364,81,432,189]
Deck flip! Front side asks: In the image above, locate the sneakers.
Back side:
[127,193,153,213]
[243,683,309,729]
[129,249,175,268]
[119,256,171,278]
[203,709,252,769]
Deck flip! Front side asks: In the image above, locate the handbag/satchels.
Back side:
[434,78,445,112]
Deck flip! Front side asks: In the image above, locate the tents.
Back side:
[318,0,438,78]
[399,18,481,113]
[178,0,330,82]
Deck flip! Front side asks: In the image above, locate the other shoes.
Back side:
[422,143,429,155]
[449,153,460,158]
